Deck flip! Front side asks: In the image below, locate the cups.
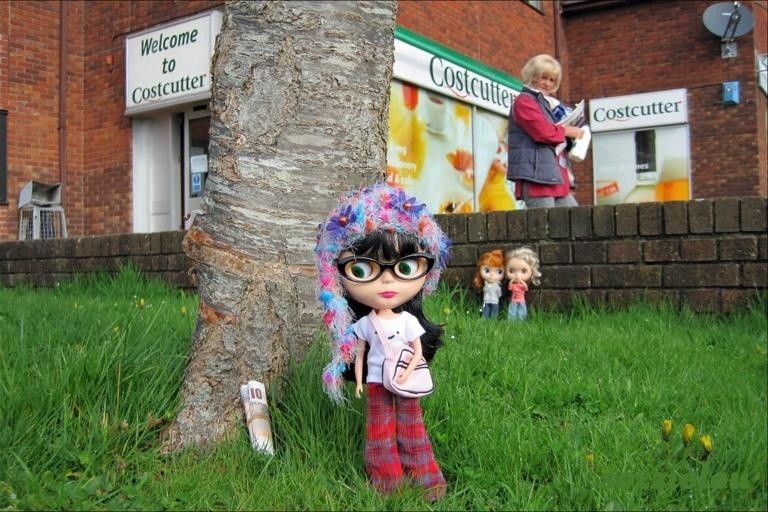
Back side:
[423,95,447,133]
[656,157,689,201]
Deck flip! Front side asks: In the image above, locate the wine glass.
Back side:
[443,129,474,210]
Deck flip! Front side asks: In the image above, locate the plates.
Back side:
[418,116,452,136]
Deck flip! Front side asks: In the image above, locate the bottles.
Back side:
[623,130,657,203]
[393,82,427,180]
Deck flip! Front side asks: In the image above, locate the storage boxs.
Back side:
[18,181,62,209]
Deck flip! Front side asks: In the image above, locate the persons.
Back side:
[315,190,451,498]
[505,54,584,208]
[503,247,539,322]
[475,251,506,322]
